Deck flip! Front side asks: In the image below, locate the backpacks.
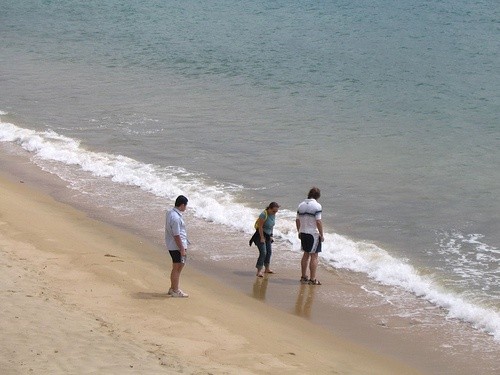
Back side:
[255,209,268,230]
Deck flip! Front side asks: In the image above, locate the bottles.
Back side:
[181,256,186,263]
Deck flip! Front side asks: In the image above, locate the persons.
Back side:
[165,195,190,297]
[249,202,280,277]
[296,187,324,285]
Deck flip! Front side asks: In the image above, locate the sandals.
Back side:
[307,279,322,285]
[301,274,308,283]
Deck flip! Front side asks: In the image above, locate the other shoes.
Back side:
[172,289,189,297]
[168,287,173,294]
